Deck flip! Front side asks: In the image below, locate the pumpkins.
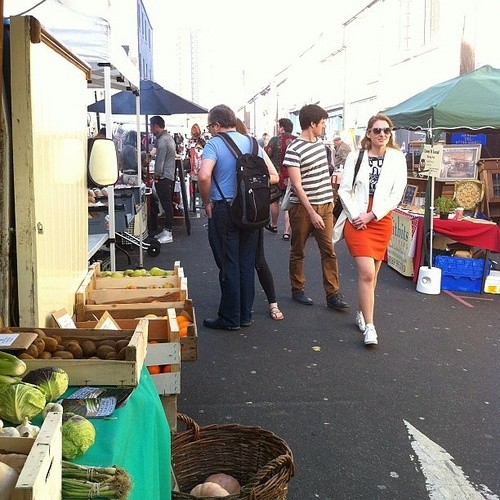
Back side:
[189,473,241,496]
[0,462,20,500]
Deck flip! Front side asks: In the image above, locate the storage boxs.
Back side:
[432,254,492,294]
[0,411,63,500]
[484,270,500,295]
[75,260,198,432]
[4,317,149,387]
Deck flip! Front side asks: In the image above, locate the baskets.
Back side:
[171,413,294,500]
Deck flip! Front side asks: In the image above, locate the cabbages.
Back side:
[0,366,95,459]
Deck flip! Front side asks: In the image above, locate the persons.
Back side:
[235,116,286,321]
[88,116,350,245]
[334,113,407,344]
[197,104,269,331]
[283,104,350,310]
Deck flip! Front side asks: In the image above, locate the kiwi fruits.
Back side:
[0,328,132,361]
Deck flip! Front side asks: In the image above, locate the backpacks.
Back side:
[210,131,270,227]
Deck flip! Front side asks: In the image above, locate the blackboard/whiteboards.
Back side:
[402,184,419,206]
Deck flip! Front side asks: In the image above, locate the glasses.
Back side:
[207,123,213,130]
[368,127,391,135]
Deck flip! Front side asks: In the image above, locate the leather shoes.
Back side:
[203,318,240,331]
[240,320,251,326]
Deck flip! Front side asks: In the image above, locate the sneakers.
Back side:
[354,311,366,333]
[363,323,378,345]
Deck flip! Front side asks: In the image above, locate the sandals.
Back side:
[283,234,290,241]
[270,307,284,320]
[265,223,277,233]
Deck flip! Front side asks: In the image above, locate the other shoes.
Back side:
[154,229,169,238]
[292,292,314,305]
[157,231,173,244]
[327,293,349,309]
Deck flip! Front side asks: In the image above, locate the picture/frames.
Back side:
[402,184,419,206]
[435,143,481,182]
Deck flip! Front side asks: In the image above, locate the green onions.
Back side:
[61,460,132,500]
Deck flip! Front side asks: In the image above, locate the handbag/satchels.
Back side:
[331,209,348,246]
[269,184,282,204]
[332,198,344,217]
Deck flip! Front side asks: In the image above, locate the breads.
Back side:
[87,188,109,230]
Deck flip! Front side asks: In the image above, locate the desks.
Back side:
[3,362,171,500]
[383,208,500,285]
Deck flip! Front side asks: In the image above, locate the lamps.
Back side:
[116,74,139,97]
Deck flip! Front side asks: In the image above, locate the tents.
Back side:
[378,65,500,269]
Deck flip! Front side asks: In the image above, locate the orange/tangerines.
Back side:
[143,315,192,373]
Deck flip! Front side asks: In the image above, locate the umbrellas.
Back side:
[87,79,208,213]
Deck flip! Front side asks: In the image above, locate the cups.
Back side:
[454,208,465,221]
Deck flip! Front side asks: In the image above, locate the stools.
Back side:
[190,176,206,213]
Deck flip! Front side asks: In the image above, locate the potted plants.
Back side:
[434,196,458,220]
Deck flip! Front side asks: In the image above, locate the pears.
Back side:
[99,267,174,278]
